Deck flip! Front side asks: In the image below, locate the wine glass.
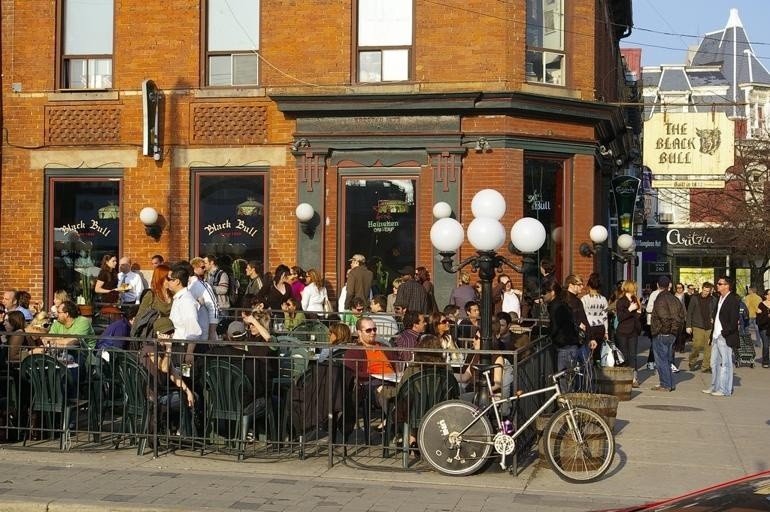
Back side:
[71,292,76,304]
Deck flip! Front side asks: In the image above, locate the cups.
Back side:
[395,362,405,381]
[274,323,283,331]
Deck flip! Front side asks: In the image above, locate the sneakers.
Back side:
[632,362,725,396]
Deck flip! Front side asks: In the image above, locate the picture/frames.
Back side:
[544,9,556,35]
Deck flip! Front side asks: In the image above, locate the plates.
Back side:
[113,288,132,292]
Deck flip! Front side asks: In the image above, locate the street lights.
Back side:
[429,186,547,387]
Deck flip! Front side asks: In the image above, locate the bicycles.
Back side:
[418,358,627,483]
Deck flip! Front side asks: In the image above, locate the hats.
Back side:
[348,254,366,263]
[153,317,176,335]
[227,321,247,338]
[398,265,414,275]
[500,276,511,285]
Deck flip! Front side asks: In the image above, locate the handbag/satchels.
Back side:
[573,319,588,346]
[600,338,627,367]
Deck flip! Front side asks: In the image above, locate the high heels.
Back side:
[374,422,395,435]
[410,442,418,457]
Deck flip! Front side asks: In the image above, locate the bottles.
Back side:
[309,335,315,357]
[34,303,40,313]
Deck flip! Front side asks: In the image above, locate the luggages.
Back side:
[733,324,756,368]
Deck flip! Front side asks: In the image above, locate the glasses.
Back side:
[34,321,52,329]
[542,289,550,295]
[167,276,176,281]
[354,306,364,312]
[718,283,727,286]
[394,307,403,310]
[360,327,377,334]
[474,334,480,340]
[286,302,293,307]
[439,319,449,324]
[199,265,205,269]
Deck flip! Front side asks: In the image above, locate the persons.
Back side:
[538,261,770,398]
[2,252,532,457]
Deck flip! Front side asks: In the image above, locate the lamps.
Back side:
[295,204,315,235]
[139,207,160,238]
[289,137,310,151]
[599,145,612,156]
[433,201,452,220]
[474,136,492,151]
